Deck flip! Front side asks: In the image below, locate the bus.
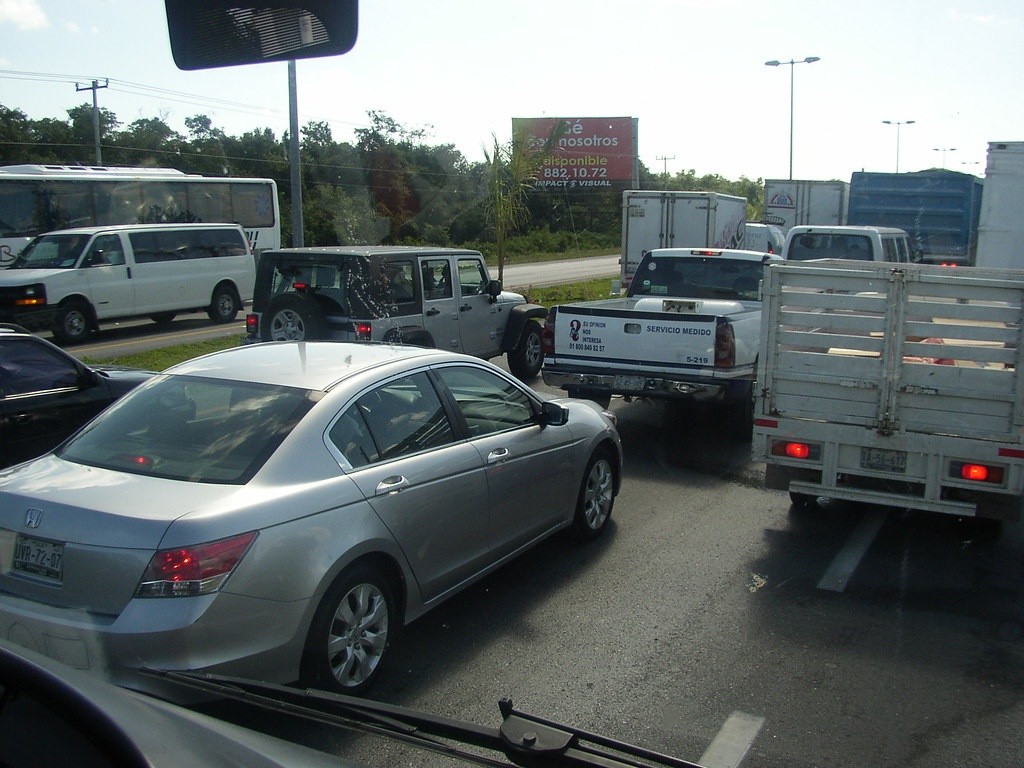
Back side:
[0,164,281,313]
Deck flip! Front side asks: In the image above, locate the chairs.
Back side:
[144,394,443,459]
[295,265,450,305]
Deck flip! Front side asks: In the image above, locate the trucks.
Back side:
[762,179,851,237]
[750,142,1024,541]
[618,190,788,292]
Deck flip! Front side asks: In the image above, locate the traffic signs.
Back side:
[512,117,633,186]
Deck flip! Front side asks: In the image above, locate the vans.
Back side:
[786,225,923,264]
[0,224,256,346]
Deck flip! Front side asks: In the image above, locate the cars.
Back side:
[0,340,623,699]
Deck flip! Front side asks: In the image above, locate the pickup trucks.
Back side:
[541,247,785,440]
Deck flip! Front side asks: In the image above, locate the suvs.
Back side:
[238,246,548,381]
[0,322,158,470]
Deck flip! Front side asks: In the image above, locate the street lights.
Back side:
[75,79,108,167]
